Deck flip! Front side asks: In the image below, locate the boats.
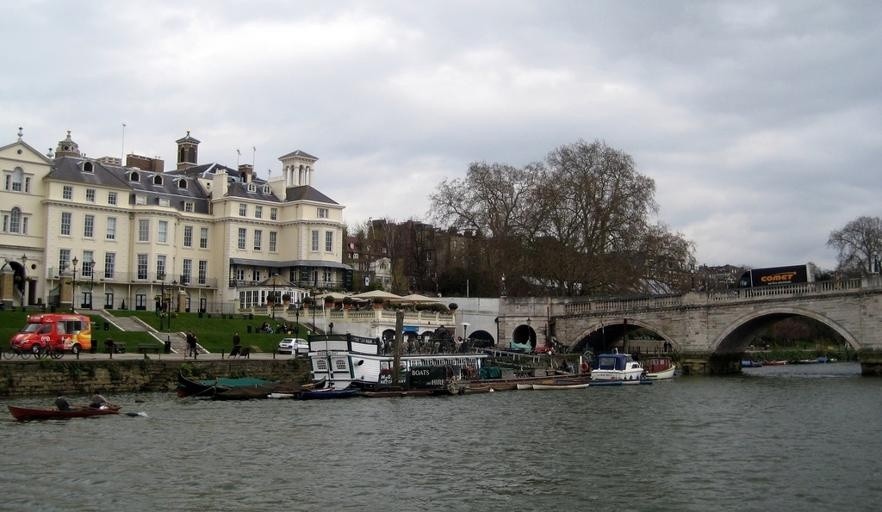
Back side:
[740,356,839,369]
[588,346,652,386]
[644,356,676,380]
[178,369,327,397]
[533,382,590,391]
[516,382,532,390]
[6,403,123,422]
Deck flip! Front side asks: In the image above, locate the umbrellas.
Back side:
[389,294,442,305]
[354,288,403,300]
[258,273,297,317]
[312,291,351,300]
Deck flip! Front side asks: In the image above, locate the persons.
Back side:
[232,330,241,347]
[186,330,193,350]
[55,392,72,410]
[90,390,107,407]
[189,335,197,357]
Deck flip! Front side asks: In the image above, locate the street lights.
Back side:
[71,256,79,314]
[20,253,27,306]
[311,284,318,332]
[159,272,166,331]
[294,297,303,338]
[171,280,178,312]
[88,259,96,310]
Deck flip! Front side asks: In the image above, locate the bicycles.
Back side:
[3,339,65,361]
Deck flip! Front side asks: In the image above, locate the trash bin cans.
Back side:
[199,313,202,319]
[165,342,170,354]
[91,340,96,354]
[104,323,109,331]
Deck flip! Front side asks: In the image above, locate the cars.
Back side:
[277,337,309,353]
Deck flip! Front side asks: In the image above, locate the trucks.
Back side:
[736,263,817,296]
[9,312,92,354]
